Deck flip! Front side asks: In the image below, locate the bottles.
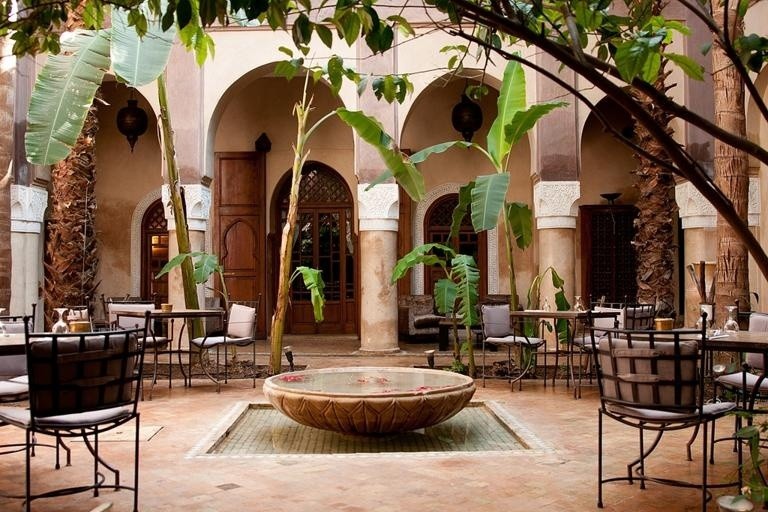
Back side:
[542,295,551,312]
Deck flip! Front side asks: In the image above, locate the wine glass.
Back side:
[50,308,70,334]
[0,307,10,337]
[724,305,740,335]
[574,295,584,313]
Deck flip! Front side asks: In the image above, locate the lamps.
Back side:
[117,88,148,153]
[451,80,484,148]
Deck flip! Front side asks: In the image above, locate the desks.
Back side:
[508,310,622,399]
[621,327,767,467]
[112,310,226,394]
[440,318,483,350]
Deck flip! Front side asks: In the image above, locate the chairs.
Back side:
[397,292,446,343]
[478,295,547,391]
[0,310,151,511]
[567,304,625,399]
[1,304,37,334]
[709,312,768,464]
[188,300,257,393]
[2,330,71,465]
[106,296,174,389]
[625,304,656,328]
[585,311,745,510]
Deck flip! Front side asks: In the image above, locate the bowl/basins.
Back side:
[656,317,674,331]
[160,304,173,313]
[70,321,90,335]
